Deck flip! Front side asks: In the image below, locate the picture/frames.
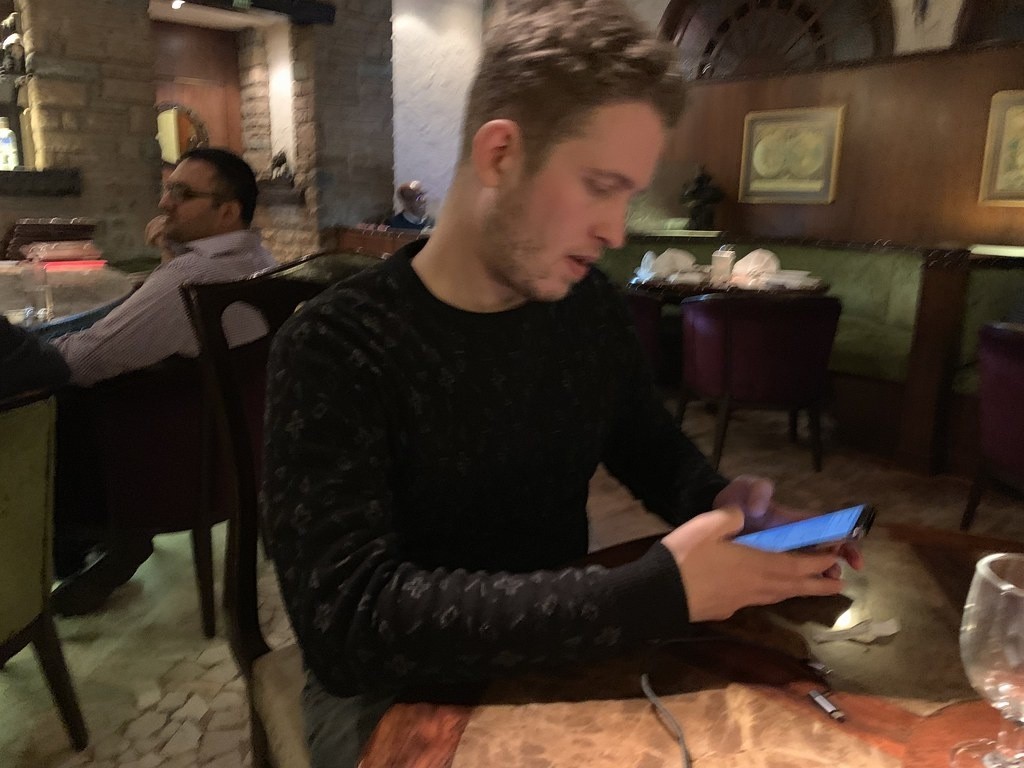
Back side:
[976,90,1024,207]
[737,104,846,205]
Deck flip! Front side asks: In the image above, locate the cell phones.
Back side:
[729,505,881,556]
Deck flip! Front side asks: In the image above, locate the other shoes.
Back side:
[49,535,154,617]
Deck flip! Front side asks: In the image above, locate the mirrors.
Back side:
[155,101,209,170]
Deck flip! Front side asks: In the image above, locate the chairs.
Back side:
[672,289,843,478]
[0,275,334,754]
[958,321,1024,530]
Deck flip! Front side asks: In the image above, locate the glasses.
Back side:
[165,185,223,203]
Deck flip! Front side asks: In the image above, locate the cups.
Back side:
[23,284,54,322]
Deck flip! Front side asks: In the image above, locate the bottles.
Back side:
[0,117,19,170]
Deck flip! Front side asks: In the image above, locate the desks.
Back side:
[346,519,1024,768]
[630,275,831,423]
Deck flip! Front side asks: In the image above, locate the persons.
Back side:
[0,148,280,622]
[253,0,866,768]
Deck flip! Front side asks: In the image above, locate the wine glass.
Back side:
[947,553,1024,768]
